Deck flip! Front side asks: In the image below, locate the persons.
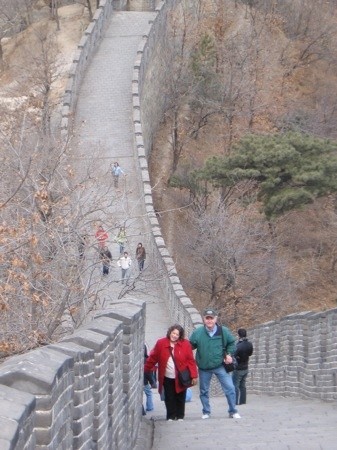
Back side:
[142,344,154,415]
[236,329,253,405]
[76,225,147,284]
[189,310,242,419]
[111,162,125,187]
[145,324,198,422]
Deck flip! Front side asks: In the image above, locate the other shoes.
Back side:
[178,419,183,422]
[168,419,173,422]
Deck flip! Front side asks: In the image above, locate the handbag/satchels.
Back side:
[178,369,194,389]
[222,356,238,372]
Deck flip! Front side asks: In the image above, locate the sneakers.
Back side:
[201,414,211,419]
[229,413,241,419]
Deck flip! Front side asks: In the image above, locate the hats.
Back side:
[203,309,218,319]
